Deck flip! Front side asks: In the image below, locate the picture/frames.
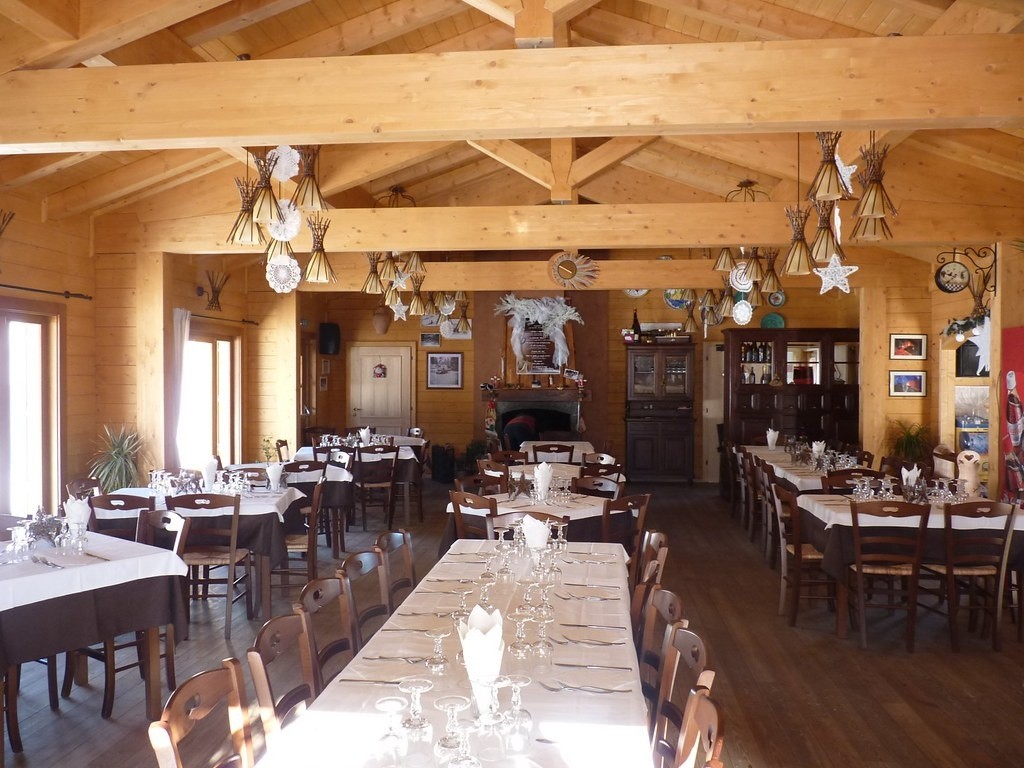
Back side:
[426,351,463,389]
[420,332,441,347]
[889,333,927,360]
[889,369,928,397]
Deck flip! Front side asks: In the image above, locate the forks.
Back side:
[30,556,52,568]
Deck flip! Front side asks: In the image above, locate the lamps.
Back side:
[226,144,339,294]
[682,127,898,338]
[203,269,232,314]
[361,185,473,336]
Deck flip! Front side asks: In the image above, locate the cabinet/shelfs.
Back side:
[718,325,859,503]
[624,336,695,485]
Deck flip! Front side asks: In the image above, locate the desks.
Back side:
[793,494,1024,644]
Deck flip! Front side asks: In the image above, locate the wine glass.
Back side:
[319,431,389,458]
[3,515,88,567]
[362,475,573,768]
[782,433,972,510]
[144,466,254,505]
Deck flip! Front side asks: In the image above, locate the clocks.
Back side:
[935,261,970,294]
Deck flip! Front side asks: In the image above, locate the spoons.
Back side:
[539,551,637,698]
[39,556,65,569]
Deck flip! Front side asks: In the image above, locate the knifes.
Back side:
[338,677,403,687]
[84,552,111,562]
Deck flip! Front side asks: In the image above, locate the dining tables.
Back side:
[438,435,1022,590]
[257,537,655,768]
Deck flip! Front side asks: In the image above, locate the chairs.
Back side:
[922,501,1020,653]
[628,529,729,768]
[771,484,857,632]
[1,424,435,767]
[845,499,932,656]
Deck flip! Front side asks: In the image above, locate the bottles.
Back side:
[740,364,746,384]
[1004,370,1023,458]
[738,339,772,364]
[1002,434,1024,510]
[630,308,642,345]
[748,366,755,384]
[760,365,768,384]
